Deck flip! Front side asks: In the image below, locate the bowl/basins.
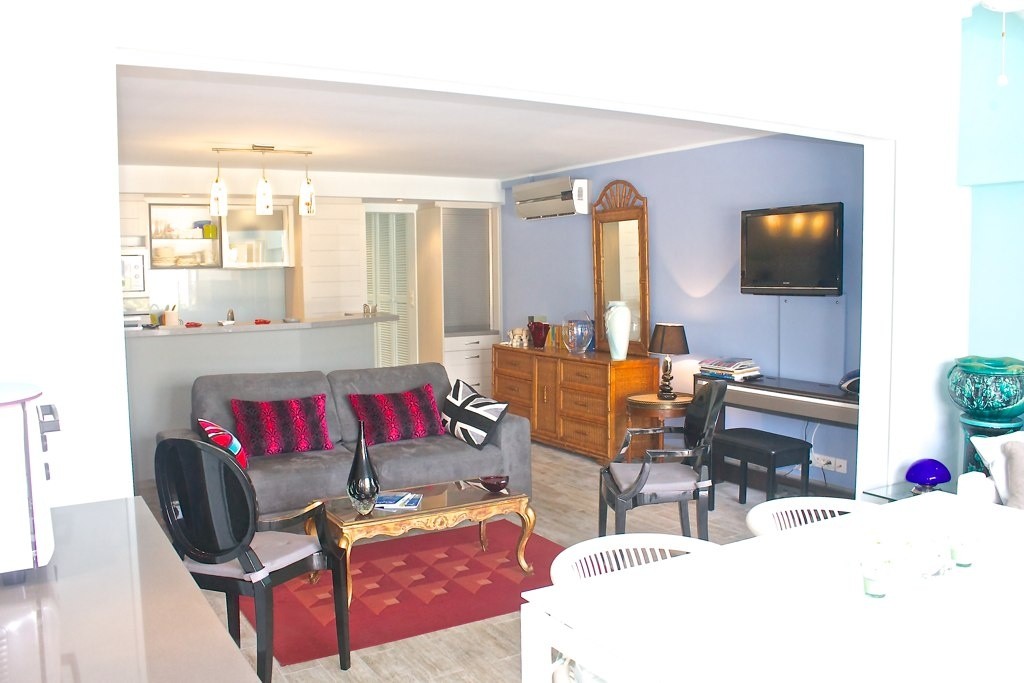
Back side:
[478,475,510,493]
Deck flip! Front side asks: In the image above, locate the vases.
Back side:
[604,301,631,361]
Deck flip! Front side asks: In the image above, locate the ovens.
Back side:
[123,297,152,327]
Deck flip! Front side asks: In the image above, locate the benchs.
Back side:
[708,428,812,511]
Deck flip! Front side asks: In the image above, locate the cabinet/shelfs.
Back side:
[148,203,223,270]
[491,344,659,464]
[443,330,499,397]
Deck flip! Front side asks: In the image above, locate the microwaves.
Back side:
[121,255,144,291]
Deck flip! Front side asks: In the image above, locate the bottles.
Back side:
[345,420,380,516]
[152,219,218,238]
[227,309,234,321]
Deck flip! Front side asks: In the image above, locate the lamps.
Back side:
[210,146,316,216]
[647,322,689,400]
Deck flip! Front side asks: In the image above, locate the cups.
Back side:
[561,310,593,355]
[205,256,215,264]
[164,310,179,327]
[204,249,215,257]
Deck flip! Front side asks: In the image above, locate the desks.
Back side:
[520,496,1024,683]
[692,373,859,431]
[863,462,963,501]
[626,391,696,464]
[0,495,262,683]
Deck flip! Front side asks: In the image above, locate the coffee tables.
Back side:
[304,479,537,608]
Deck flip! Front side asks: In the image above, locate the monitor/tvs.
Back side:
[740,202,845,295]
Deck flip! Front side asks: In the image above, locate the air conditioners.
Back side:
[511,176,589,220]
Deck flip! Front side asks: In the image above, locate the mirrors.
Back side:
[592,180,650,356]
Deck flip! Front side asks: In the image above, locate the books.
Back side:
[699,357,760,382]
[375,492,422,511]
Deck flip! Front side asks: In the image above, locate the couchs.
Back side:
[156,362,532,515]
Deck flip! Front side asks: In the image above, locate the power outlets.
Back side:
[810,454,847,473]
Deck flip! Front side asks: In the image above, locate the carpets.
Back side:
[237,519,566,666]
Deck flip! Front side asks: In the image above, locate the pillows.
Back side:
[230,394,335,457]
[347,384,447,447]
[441,379,510,450]
[970,431,1024,505]
[196,418,248,471]
[1002,441,1024,509]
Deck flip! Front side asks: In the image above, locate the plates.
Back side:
[152,247,217,266]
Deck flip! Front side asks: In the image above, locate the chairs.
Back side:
[550,533,721,586]
[600,374,727,541]
[155,439,351,683]
[746,496,878,537]
